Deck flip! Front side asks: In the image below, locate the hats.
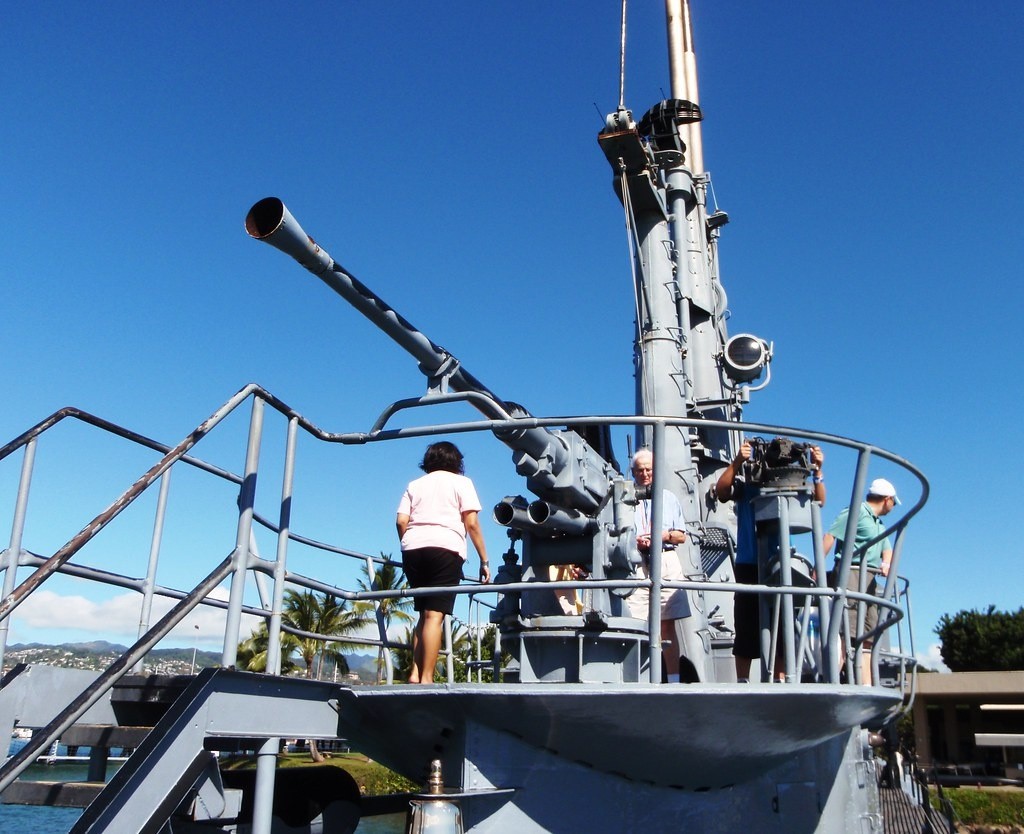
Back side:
[868,481,901,505]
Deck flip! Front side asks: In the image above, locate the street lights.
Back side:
[191,623,200,676]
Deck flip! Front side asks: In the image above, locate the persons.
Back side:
[811,478,901,687]
[396,442,490,685]
[624,449,692,684]
[716,437,825,685]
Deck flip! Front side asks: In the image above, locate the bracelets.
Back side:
[669,532,673,541]
[881,563,890,569]
[481,561,489,567]
[813,475,824,484]
